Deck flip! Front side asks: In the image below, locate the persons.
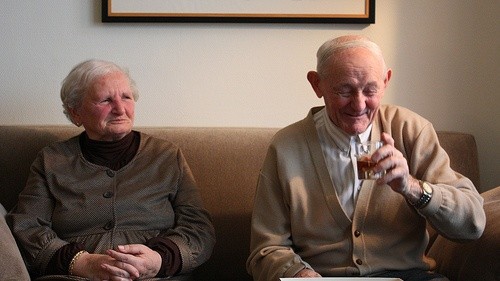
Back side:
[246,35,486,281]
[8,59,215,281]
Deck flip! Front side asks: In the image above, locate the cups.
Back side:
[350,141,387,180]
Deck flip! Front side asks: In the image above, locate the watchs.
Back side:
[408,180,433,210]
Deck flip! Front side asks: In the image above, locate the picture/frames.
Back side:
[101,0,376,23]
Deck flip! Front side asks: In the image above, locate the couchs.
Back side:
[0,123,500,281]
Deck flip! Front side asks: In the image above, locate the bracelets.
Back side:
[69,250,89,275]
